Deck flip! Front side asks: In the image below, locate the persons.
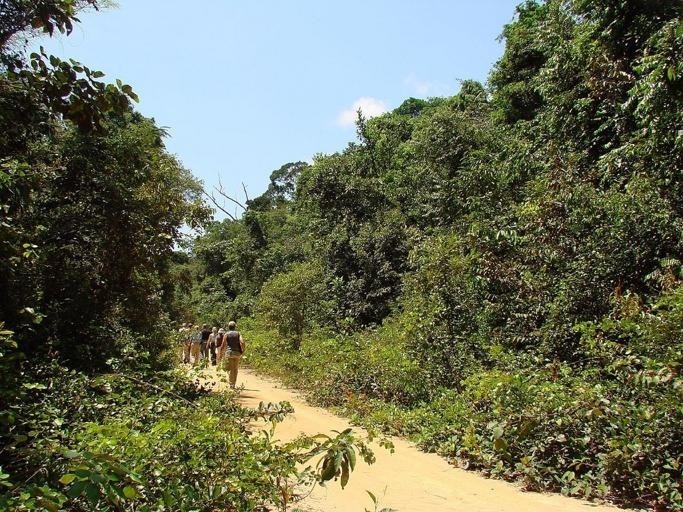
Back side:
[179,323,224,366]
[219,321,244,390]
